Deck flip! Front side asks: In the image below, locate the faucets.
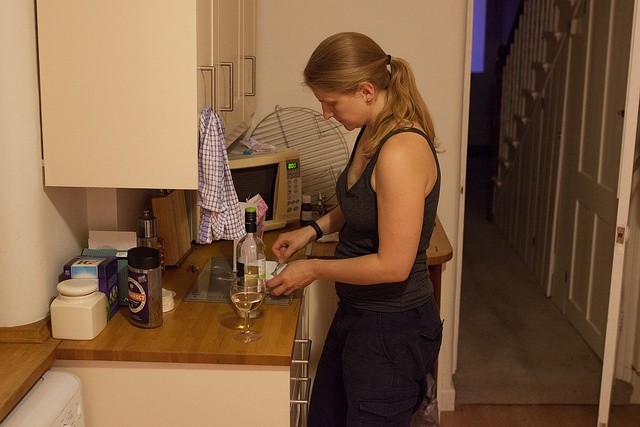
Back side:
[317,191,326,214]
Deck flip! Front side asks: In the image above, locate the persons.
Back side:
[265,32,446,426]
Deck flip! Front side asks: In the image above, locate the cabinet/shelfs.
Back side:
[53,256,310,423]
[36,1,263,190]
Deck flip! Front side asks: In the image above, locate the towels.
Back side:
[197,107,246,244]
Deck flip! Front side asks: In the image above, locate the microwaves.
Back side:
[190,149,302,244]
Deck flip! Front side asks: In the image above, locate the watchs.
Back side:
[307,219,324,240]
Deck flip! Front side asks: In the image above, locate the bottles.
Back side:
[300,195,313,226]
[236,206,266,299]
[126,246,164,329]
[49,278,107,340]
[137,208,157,245]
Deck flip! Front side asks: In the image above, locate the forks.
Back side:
[271,261,286,277]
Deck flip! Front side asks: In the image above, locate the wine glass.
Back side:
[229,277,265,344]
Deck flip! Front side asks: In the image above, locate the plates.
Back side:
[266,260,288,283]
